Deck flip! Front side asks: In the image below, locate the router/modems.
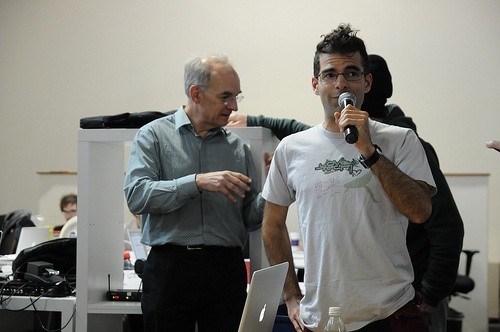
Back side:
[106,274,145,302]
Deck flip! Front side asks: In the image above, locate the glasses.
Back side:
[316,68,366,82]
[62,210,76,217]
[199,86,244,105]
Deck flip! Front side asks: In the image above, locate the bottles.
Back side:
[124,252,133,270]
[324,306,346,332]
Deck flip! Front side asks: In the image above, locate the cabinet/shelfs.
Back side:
[77,126,273,332]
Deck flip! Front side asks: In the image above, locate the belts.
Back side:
[185,243,219,251]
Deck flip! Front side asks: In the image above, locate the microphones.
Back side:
[337,92,359,144]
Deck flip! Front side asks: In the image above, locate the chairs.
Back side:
[0,209,36,255]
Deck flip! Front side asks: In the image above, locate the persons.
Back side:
[53,195,78,232]
[226,23,464,332]
[124,53,273,332]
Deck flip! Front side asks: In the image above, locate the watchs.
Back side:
[358,143,382,170]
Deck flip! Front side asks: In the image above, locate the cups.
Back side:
[244,259,250,284]
[290,233,299,251]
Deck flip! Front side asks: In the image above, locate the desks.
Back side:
[0,295,76,332]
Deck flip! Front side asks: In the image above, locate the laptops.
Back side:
[238,261,289,332]
[127,229,148,260]
[0,226,50,260]
